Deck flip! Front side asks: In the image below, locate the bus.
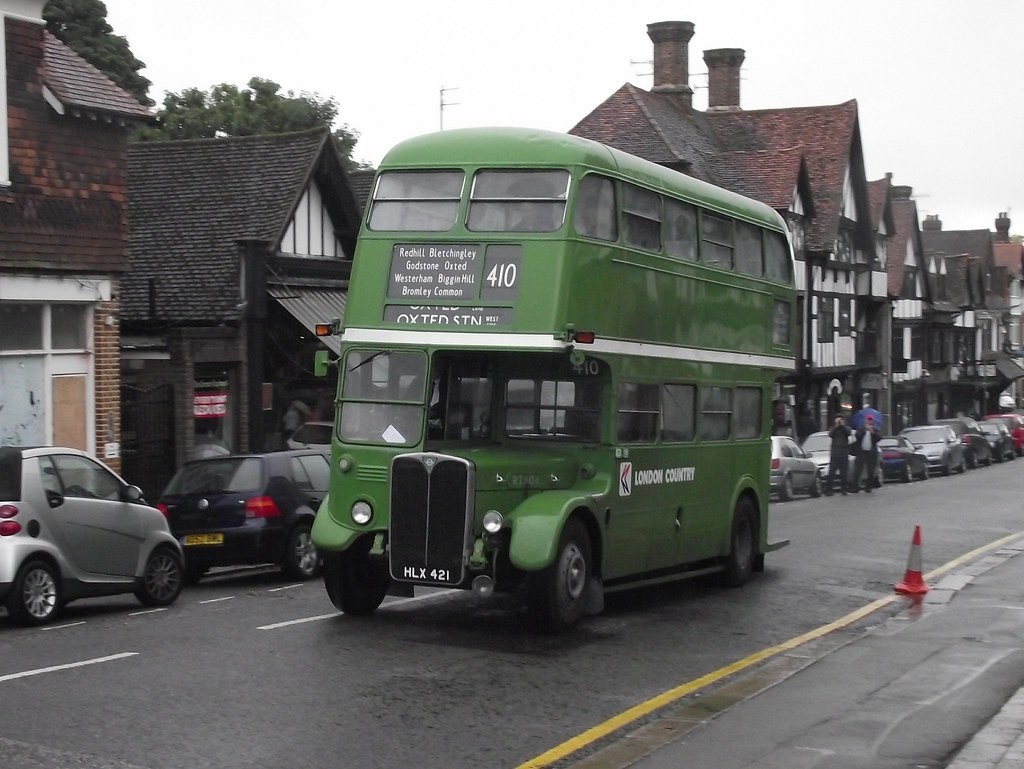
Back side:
[308,127,806,644]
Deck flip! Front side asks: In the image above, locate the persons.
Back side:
[427,376,471,436]
[851,414,881,493]
[284,406,301,439]
[826,413,852,495]
[510,203,554,231]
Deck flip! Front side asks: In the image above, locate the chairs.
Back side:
[562,408,629,444]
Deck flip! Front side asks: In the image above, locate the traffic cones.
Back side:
[895,524,929,596]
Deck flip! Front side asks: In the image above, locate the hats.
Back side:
[865,413,874,420]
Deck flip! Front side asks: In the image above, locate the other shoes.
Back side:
[841,490,848,495]
[866,486,872,493]
[826,491,834,496]
[848,488,859,493]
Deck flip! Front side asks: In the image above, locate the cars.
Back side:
[974,418,1015,466]
[894,424,968,477]
[1,445,186,631]
[870,435,932,484]
[154,453,345,592]
[982,413,1024,456]
[927,416,992,470]
[801,429,883,494]
[760,435,821,501]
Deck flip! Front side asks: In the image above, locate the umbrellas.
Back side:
[850,409,883,429]
